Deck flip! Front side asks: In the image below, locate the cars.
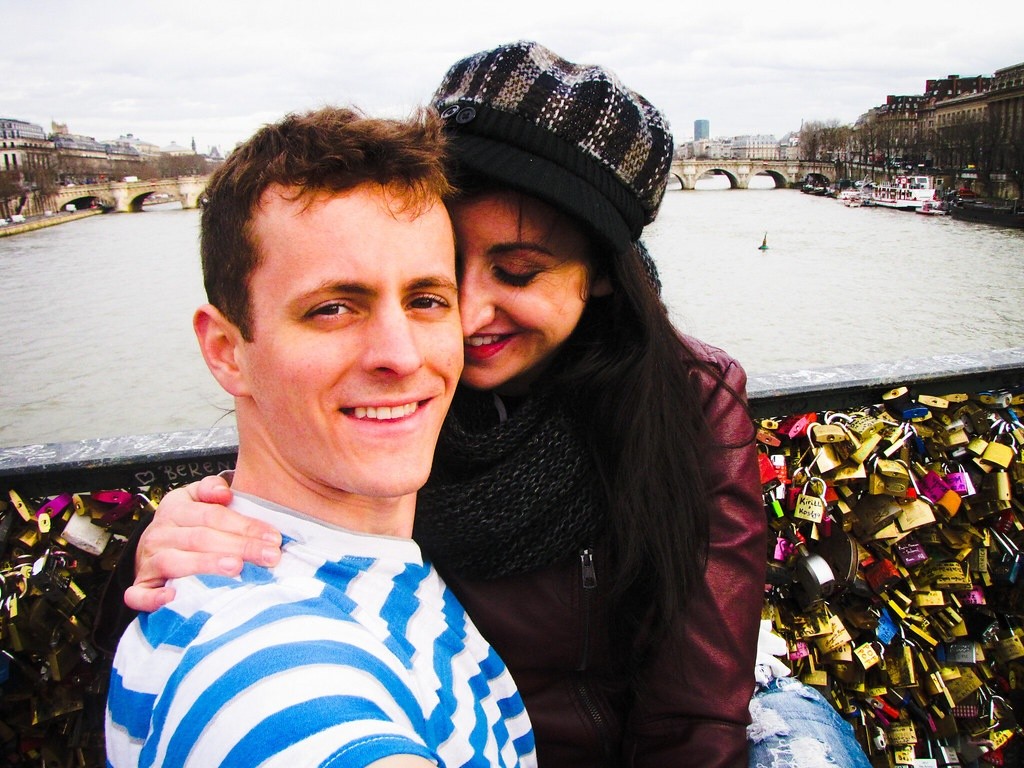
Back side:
[91,204,102,209]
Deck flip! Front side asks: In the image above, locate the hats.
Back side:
[426,41,675,255]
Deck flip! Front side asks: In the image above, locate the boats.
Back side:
[950,197,1024,227]
[802,175,949,216]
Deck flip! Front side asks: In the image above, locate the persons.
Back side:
[103,105,539,768]
[123,39,874,768]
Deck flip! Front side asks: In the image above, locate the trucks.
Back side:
[11,215,26,223]
[66,204,77,212]
[121,176,138,183]
[0,219,8,227]
